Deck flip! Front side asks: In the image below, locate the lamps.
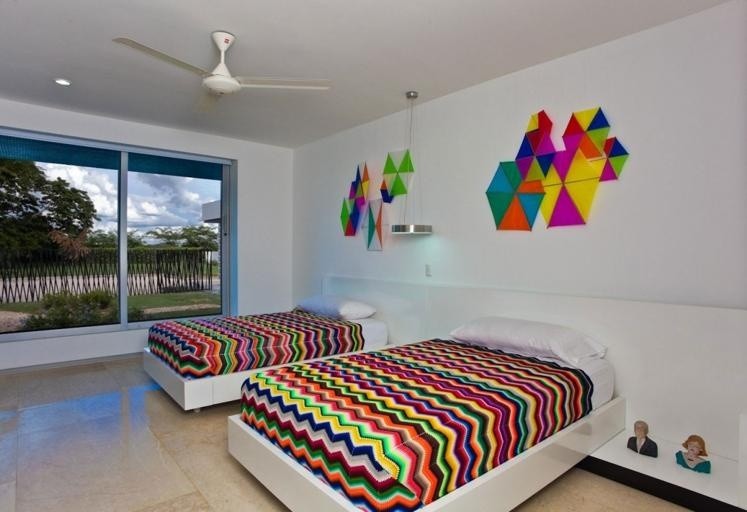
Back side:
[391,91,432,235]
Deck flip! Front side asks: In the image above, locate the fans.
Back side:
[111,31,330,114]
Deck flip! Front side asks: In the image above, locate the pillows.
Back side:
[438,315,609,370]
[296,294,377,321]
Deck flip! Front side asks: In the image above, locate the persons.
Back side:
[627,421,658,458]
[677,435,711,473]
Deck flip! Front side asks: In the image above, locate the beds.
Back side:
[228,333,626,512]
[143,310,388,413]
